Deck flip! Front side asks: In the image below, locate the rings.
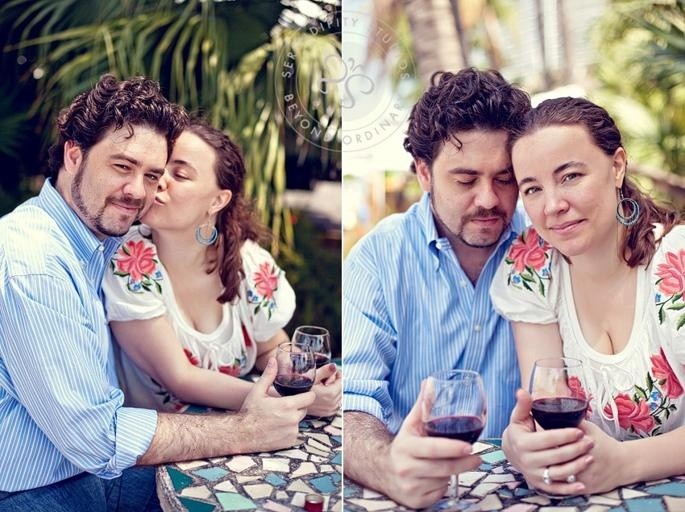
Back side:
[338,402,341,412]
[543,468,552,486]
[566,476,575,482]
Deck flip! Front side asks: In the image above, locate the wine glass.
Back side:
[290,326,331,368]
[273,343,316,397]
[423,369,488,511]
[528,357,590,430]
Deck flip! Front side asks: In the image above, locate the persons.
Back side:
[103,118,340,422]
[1,78,316,512]
[341,66,533,507]
[486,96,685,500]
